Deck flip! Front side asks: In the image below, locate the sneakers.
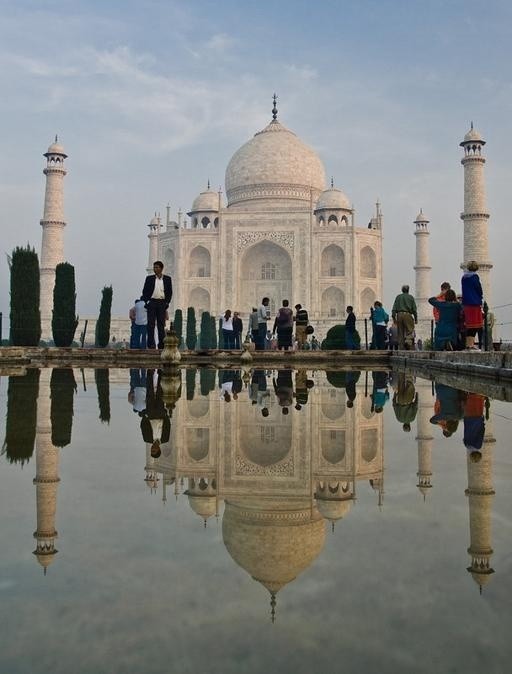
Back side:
[448,342,455,350]
[468,345,482,352]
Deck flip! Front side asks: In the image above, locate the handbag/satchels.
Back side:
[307,326,314,334]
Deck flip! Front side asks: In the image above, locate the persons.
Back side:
[222,299,318,352]
[217,367,309,417]
[130,261,172,351]
[344,306,356,350]
[128,367,172,458]
[374,285,418,350]
[432,382,486,463]
[429,260,489,352]
[371,370,420,432]
[345,369,357,408]
